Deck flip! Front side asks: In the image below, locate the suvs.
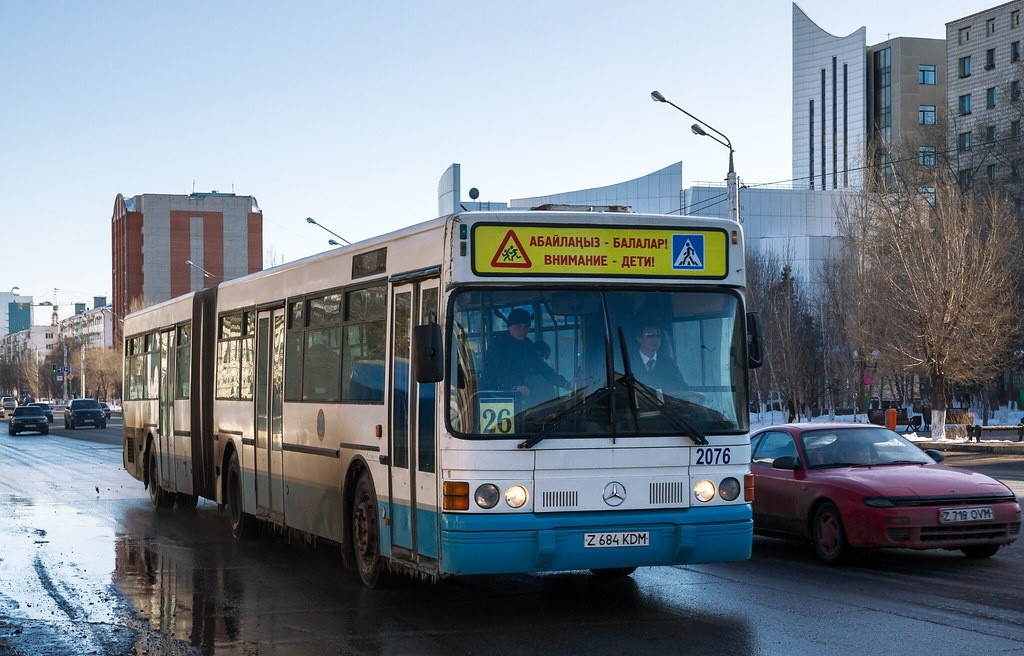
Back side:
[62,398,107,430]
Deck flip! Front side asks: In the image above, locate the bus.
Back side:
[120,204,765,590]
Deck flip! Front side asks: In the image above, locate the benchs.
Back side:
[904,414,932,433]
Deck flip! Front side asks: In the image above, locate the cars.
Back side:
[0,393,36,418]
[749,421,1022,569]
[99,402,111,419]
[26,402,55,424]
[8,405,52,436]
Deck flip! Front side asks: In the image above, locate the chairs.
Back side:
[783,440,799,456]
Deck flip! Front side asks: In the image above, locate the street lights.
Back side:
[62,323,86,398]
[27,341,40,403]
[652,89,741,224]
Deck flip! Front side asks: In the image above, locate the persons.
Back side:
[622,320,697,402]
[483,308,583,411]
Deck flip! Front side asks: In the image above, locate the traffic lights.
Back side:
[52,364,57,373]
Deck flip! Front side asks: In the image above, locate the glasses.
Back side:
[643,331,662,338]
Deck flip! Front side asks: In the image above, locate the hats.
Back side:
[507,309,531,328]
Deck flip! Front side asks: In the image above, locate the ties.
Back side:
[646,359,655,372]
